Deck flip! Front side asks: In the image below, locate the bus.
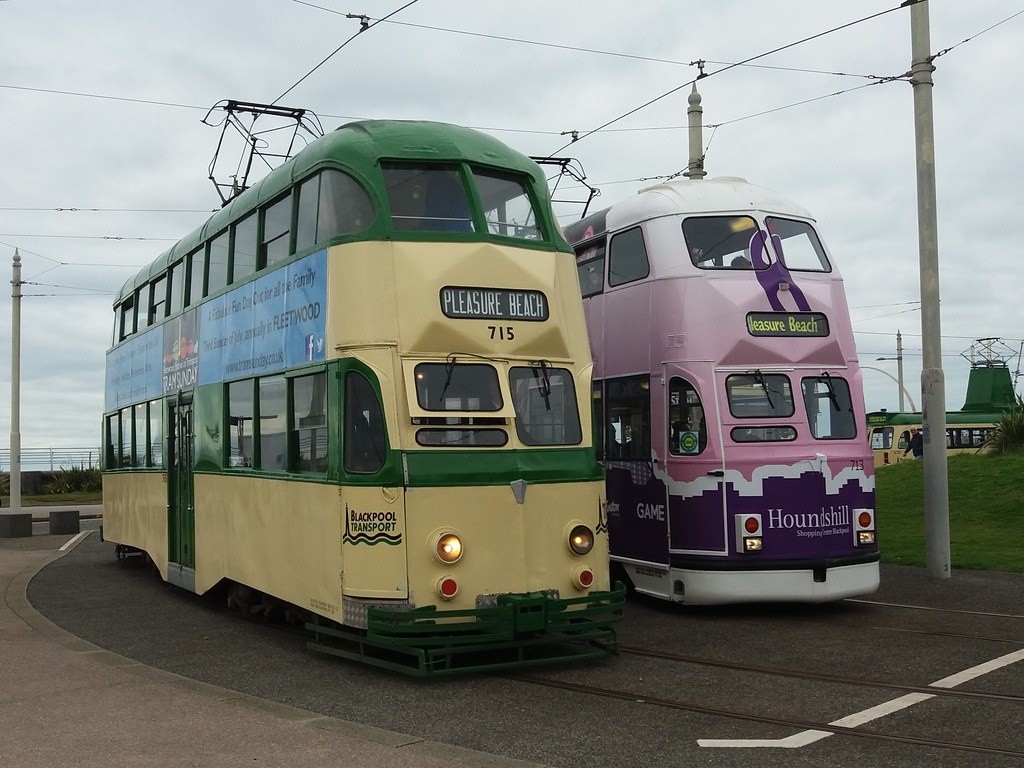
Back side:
[561,175,881,604]
[101,119,628,679]
[866,367,1023,468]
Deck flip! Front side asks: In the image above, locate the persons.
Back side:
[902,426,923,460]
